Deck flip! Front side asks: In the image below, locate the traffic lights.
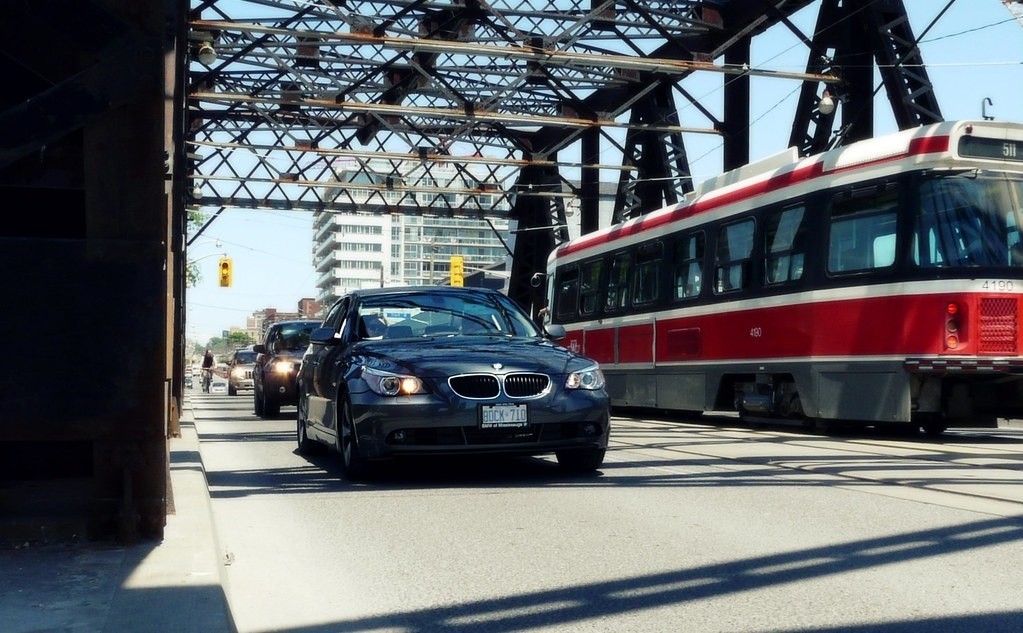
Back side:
[218,258,232,287]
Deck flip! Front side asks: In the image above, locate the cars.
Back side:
[291,284,614,484]
[248,318,326,418]
[227,348,262,397]
[183,362,230,388]
[211,381,228,393]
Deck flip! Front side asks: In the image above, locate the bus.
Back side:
[530,96,1023,445]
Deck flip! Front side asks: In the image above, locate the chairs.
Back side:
[280,334,298,348]
[359,312,496,338]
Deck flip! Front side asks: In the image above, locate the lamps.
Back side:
[198,41,217,65]
[192,183,203,200]
[565,201,573,216]
[818,90,833,114]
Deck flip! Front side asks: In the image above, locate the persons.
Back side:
[367,313,388,337]
[199,349,217,383]
[538,305,548,317]
